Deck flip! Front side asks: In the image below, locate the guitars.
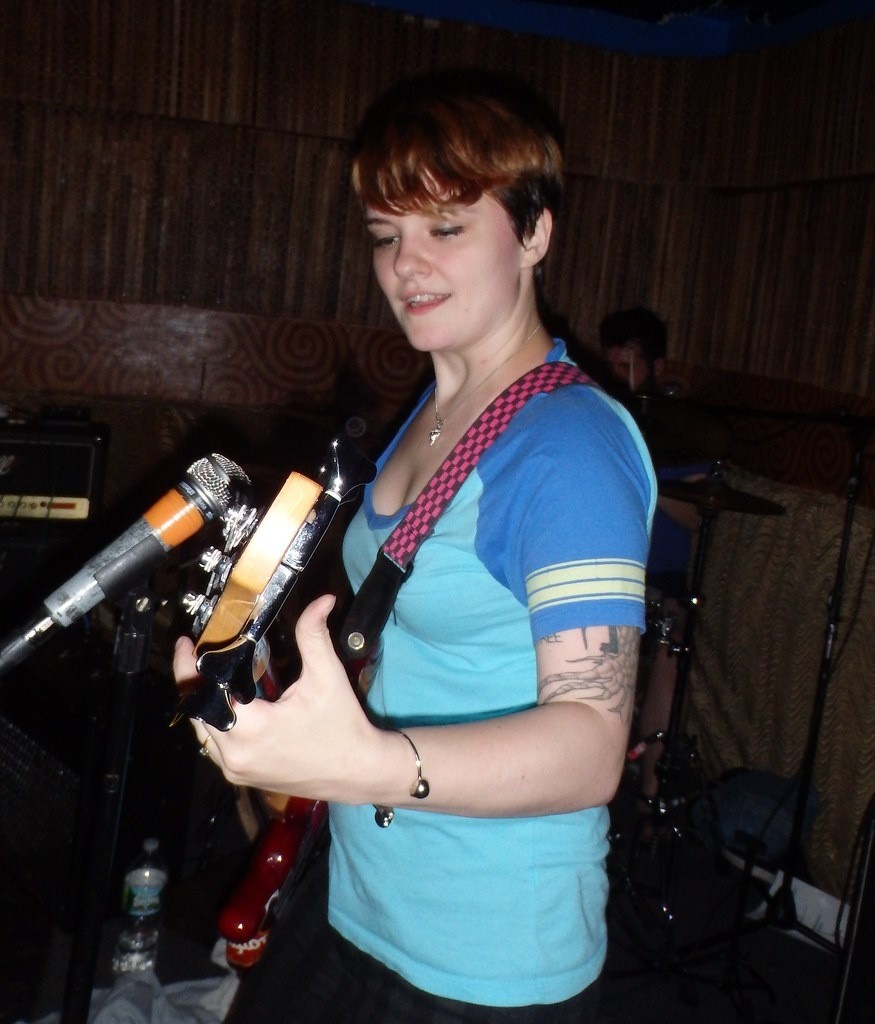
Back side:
[182,467,380,970]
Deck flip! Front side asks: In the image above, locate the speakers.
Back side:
[0,689,198,931]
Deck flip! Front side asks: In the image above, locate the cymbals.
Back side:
[654,474,788,520]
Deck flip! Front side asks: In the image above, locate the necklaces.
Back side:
[428,321,541,446]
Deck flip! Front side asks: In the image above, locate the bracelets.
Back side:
[373,728,431,827]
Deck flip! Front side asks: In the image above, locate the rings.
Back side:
[200,734,211,757]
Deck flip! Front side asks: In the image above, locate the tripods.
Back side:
[604,391,875,983]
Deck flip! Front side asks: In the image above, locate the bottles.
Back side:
[113,836,168,976]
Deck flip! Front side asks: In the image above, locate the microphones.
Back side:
[0,453,252,675]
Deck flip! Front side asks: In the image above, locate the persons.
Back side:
[173,68,658,1024]
[601,307,724,812]
